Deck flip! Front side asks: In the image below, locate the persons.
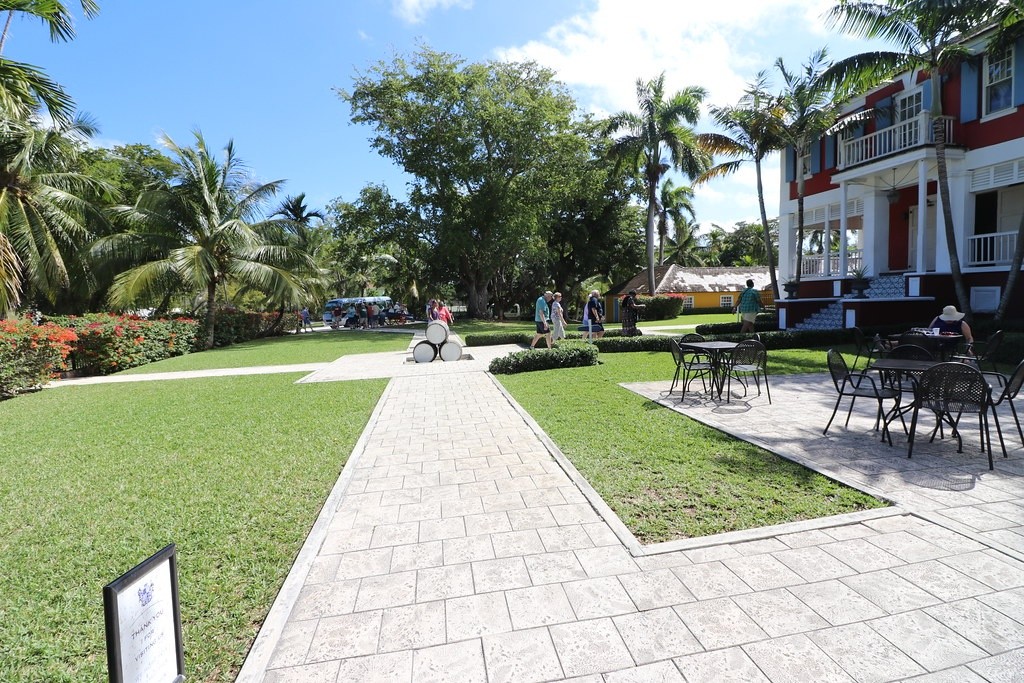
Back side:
[732,278,768,338]
[622,290,646,331]
[287,295,456,341]
[929,305,974,363]
[529,290,554,350]
[550,292,568,343]
[581,289,607,344]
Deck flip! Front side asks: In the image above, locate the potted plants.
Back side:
[847,265,873,299]
[780,276,802,299]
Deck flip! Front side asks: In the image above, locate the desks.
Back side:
[872,357,984,453]
[891,332,962,381]
[681,342,754,403]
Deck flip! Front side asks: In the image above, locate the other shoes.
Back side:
[528,347,535,350]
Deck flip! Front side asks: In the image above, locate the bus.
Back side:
[323,296,393,329]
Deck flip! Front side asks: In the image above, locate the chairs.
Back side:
[823,327,1024,470]
[670,332,772,404]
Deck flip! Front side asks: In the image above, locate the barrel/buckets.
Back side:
[413,340,437,363]
[439,341,462,361]
[426,319,449,344]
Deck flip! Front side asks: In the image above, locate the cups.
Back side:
[933,328,940,335]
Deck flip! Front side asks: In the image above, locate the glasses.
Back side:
[557,296,562,297]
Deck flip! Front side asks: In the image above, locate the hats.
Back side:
[440,301,444,307]
[588,294,593,297]
[939,305,966,321]
